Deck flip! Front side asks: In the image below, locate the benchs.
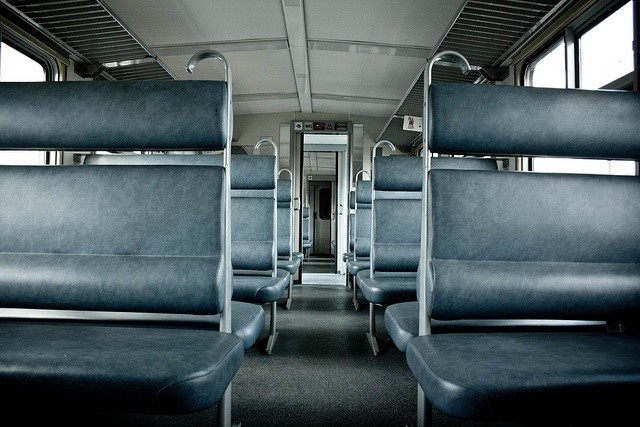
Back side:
[0,49,243,425]
[343,170,423,311]
[346,181,357,289]
[81,139,286,355]
[277,167,299,309]
[356,140,512,364]
[300,200,311,258]
[406,49,640,422]
